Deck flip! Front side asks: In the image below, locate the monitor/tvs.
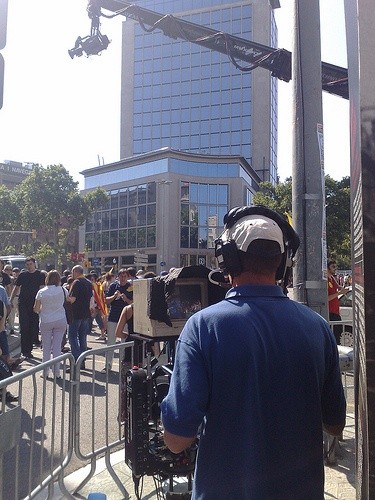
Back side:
[133,278,209,336]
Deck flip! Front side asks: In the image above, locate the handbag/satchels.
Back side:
[61,286,74,324]
[89,285,97,318]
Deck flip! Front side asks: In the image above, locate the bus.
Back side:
[0,255,39,272]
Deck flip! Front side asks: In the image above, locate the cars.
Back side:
[337,285,354,347]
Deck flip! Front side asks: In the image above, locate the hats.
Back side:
[217,214,286,257]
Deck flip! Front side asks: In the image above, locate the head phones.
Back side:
[214,205,299,280]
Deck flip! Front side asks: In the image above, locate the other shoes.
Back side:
[101,367,111,374]
[8,357,27,369]
[81,362,85,368]
[87,347,92,350]
[40,373,49,379]
[66,367,71,372]
[0,391,19,402]
[61,346,70,353]
[10,329,14,334]
[95,335,106,340]
[55,374,61,378]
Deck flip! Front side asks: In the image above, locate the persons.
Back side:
[66,264,92,372]
[0,299,18,402]
[114,302,159,426]
[1,285,25,371]
[160,214,346,500]
[327,259,349,345]
[100,268,133,374]
[333,273,352,291]
[32,270,69,381]
[0,258,175,357]
